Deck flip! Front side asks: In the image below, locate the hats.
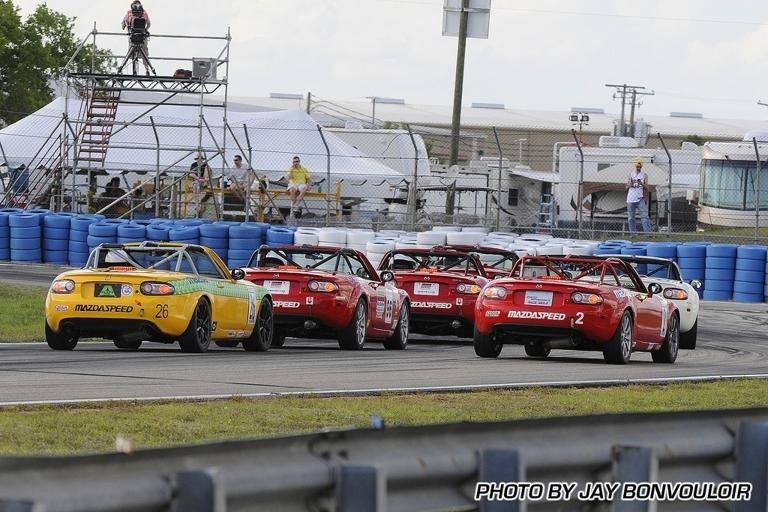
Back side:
[195,153,206,160]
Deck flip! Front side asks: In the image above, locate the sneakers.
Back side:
[291,204,302,213]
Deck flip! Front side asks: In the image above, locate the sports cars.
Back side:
[43,241,274,354]
[231,243,412,350]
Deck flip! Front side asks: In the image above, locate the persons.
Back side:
[121,0,152,74]
[188,154,211,191]
[226,154,256,195]
[284,156,311,217]
[132,180,146,198]
[98,182,117,203]
[108,176,127,204]
[622,159,655,237]
